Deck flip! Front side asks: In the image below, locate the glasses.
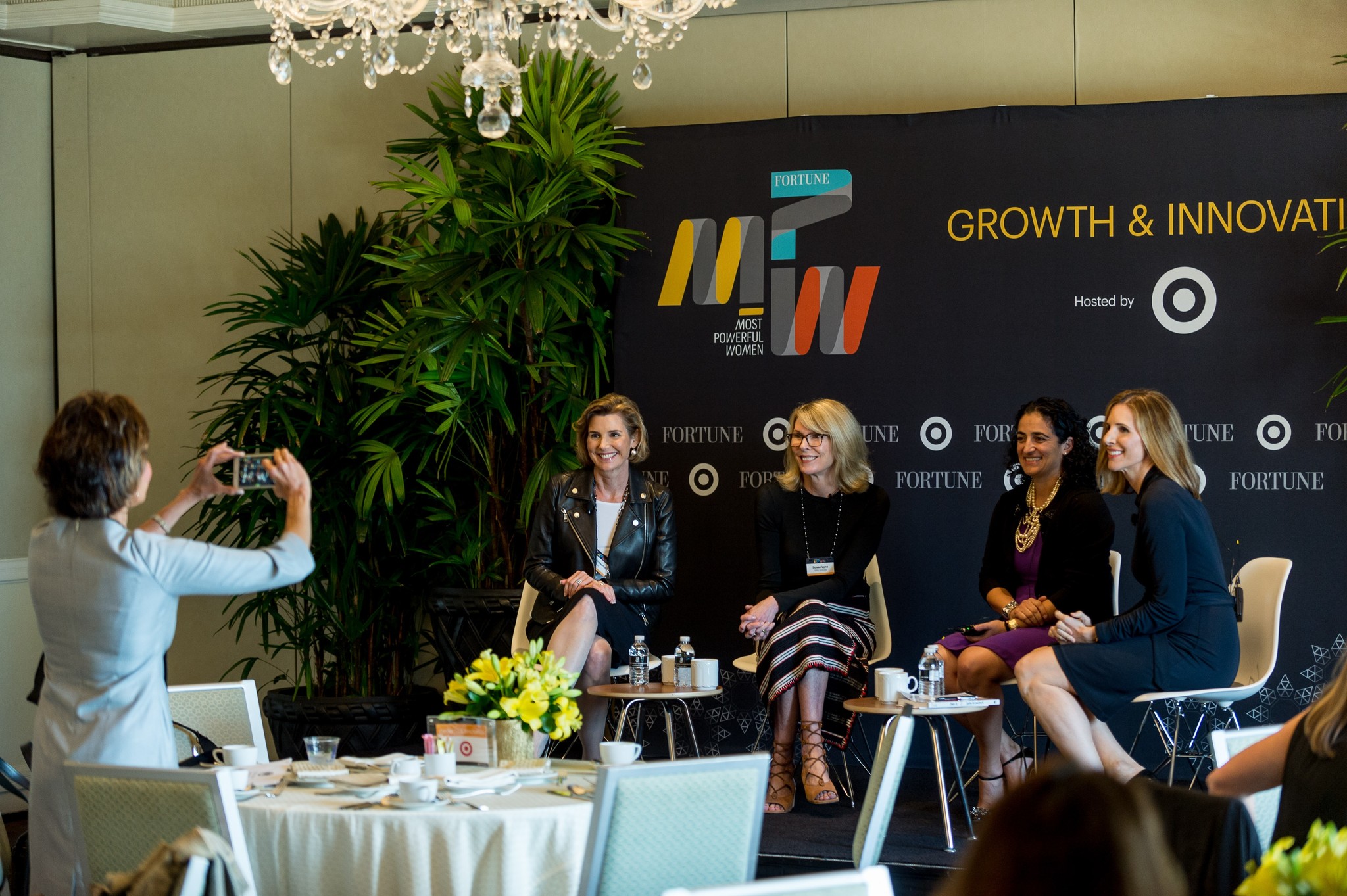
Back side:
[787,433,830,448]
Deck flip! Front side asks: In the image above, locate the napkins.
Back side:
[341,752,423,766]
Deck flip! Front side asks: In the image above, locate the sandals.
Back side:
[970,772,1005,823]
[1003,745,1042,767]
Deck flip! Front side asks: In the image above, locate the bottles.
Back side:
[674,636,695,687]
[928,645,945,695]
[629,635,649,687]
[918,648,938,695]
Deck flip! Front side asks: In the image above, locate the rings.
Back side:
[762,630,766,633]
[754,633,757,636]
[575,579,582,585]
[598,582,605,586]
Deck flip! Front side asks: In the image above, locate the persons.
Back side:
[943,763,1187,896]
[739,399,890,813]
[523,393,676,762]
[1206,656,1347,855]
[922,396,1115,822]
[1015,390,1241,784]
[28,391,316,896]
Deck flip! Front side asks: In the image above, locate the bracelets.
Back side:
[1002,601,1019,618]
[1008,618,1019,630]
[1004,621,1010,632]
[151,514,170,534]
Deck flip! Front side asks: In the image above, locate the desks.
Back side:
[843,696,997,853]
[231,758,601,896]
[587,682,724,763]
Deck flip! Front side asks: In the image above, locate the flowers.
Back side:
[437,636,584,740]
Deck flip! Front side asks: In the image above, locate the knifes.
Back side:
[547,789,595,803]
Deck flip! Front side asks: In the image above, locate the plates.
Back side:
[381,791,452,808]
[515,770,559,785]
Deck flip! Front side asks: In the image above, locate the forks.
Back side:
[436,793,489,810]
[236,779,288,802]
[452,782,522,799]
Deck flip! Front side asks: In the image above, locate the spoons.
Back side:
[341,794,398,810]
[567,785,595,799]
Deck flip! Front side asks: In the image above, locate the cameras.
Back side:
[232,452,276,490]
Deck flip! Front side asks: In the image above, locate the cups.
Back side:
[875,668,904,700]
[661,655,674,685]
[390,755,422,779]
[690,658,718,690]
[424,753,456,783]
[303,736,340,763]
[212,745,257,766]
[600,741,642,765]
[879,671,918,704]
[399,779,439,803]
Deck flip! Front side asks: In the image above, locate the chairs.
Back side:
[731,553,893,809]
[1128,555,1292,790]
[63,759,258,896]
[511,577,541,658]
[167,680,269,766]
[577,751,771,896]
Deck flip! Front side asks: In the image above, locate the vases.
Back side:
[495,719,534,761]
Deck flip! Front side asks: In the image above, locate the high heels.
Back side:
[799,721,839,804]
[764,740,796,813]
